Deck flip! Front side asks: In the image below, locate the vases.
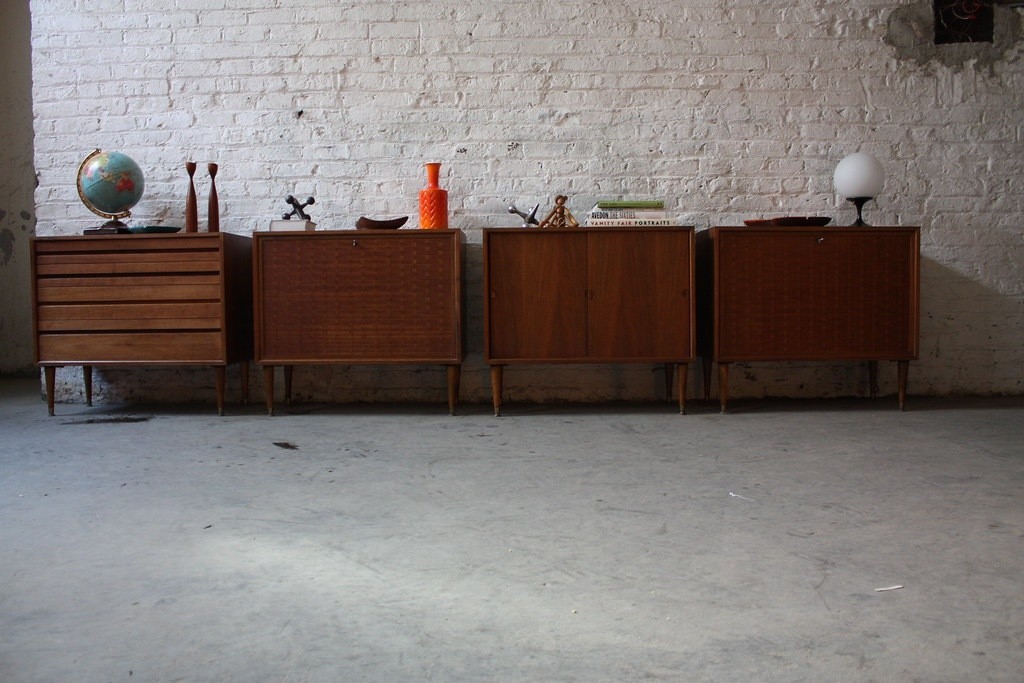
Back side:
[419,163,449,229]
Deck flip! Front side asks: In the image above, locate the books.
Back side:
[585,200,675,227]
[270,219,317,231]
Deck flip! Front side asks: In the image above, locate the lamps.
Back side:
[833,152,885,226]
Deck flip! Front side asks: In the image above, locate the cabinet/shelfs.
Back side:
[253,228,467,417]
[482,224,697,415]
[697,225,922,417]
[29,231,253,417]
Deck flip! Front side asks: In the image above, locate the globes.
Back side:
[76,148,145,235]
